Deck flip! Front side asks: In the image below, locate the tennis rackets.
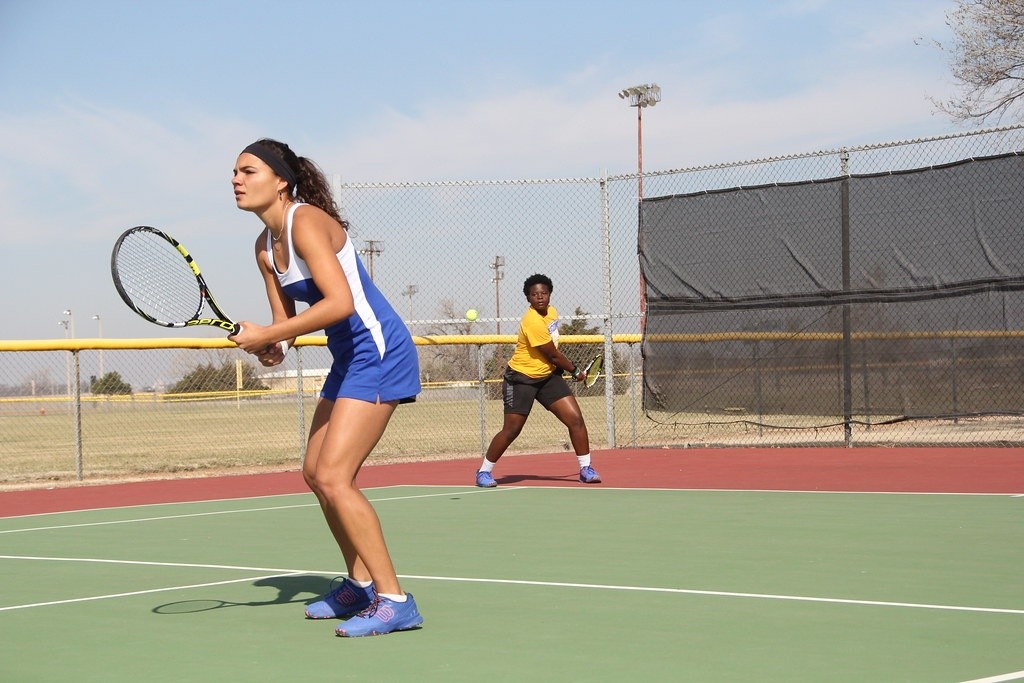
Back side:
[109,226,280,360]
[571,354,604,388]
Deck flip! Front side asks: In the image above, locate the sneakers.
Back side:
[474,468,498,487]
[335,586,425,638]
[579,464,602,483]
[304,576,377,619]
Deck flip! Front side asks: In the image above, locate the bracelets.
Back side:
[280,341,289,357]
[572,366,580,377]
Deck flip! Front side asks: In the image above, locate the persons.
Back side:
[475,274,603,487]
[229,140,424,636]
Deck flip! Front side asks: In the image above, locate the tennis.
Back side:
[466,309,478,321]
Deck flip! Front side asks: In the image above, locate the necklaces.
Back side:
[272,202,291,241]
